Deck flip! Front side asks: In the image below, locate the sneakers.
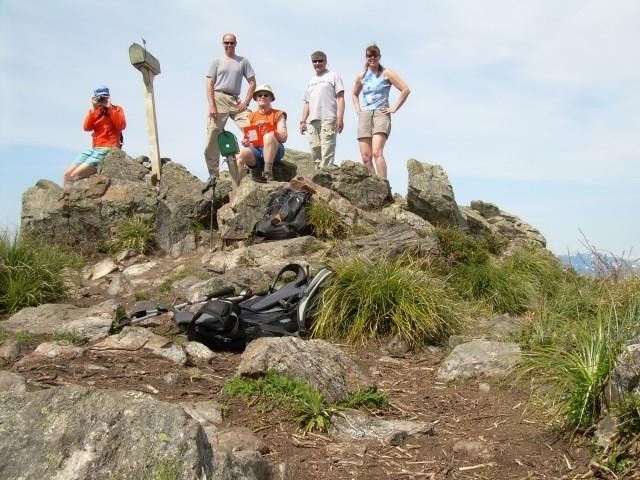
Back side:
[263,172,273,181]
[208,177,220,186]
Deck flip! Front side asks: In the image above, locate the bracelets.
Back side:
[299,121,308,126]
[243,99,250,106]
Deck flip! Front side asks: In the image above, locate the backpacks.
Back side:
[174,263,335,349]
[255,187,313,238]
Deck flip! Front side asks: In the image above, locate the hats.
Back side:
[94,87,109,96]
[253,84,275,101]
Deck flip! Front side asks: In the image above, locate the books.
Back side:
[241,121,279,148]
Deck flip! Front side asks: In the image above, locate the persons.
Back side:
[351,44,412,179]
[236,83,288,181]
[203,33,258,180]
[298,51,347,170]
[62,86,127,186]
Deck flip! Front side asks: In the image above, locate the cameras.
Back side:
[96,96,105,103]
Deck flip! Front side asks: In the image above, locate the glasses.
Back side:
[258,94,268,97]
[224,42,235,45]
[312,60,323,63]
[368,55,378,58]
